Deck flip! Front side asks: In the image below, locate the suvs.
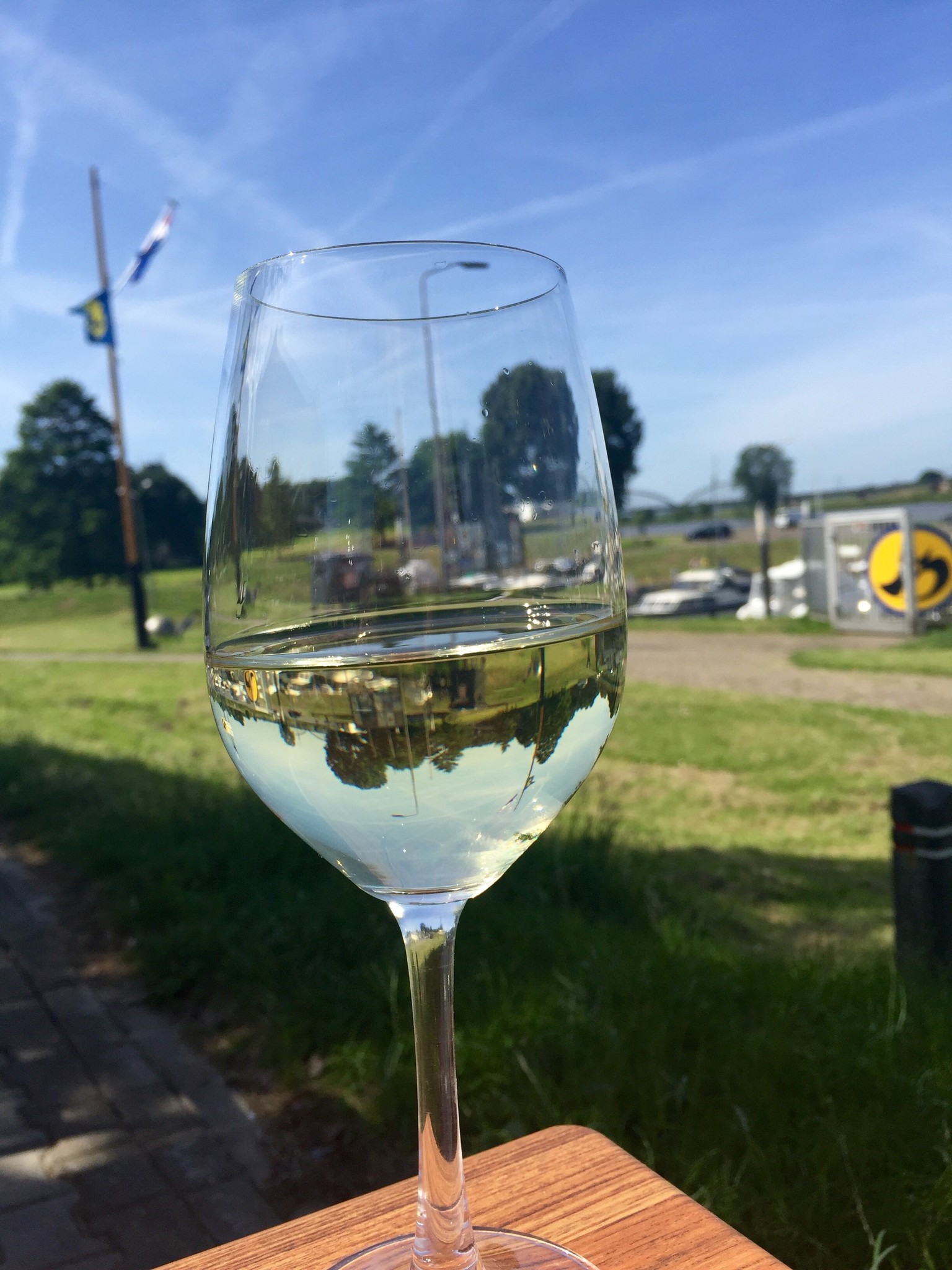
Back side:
[628,559,752,624]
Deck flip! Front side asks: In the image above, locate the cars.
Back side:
[302,549,606,614]
[686,518,735,543]
[736,542,875,630]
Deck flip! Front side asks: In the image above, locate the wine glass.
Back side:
[205,240,627,1268]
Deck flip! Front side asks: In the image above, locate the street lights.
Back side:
[418,257,491,572]
